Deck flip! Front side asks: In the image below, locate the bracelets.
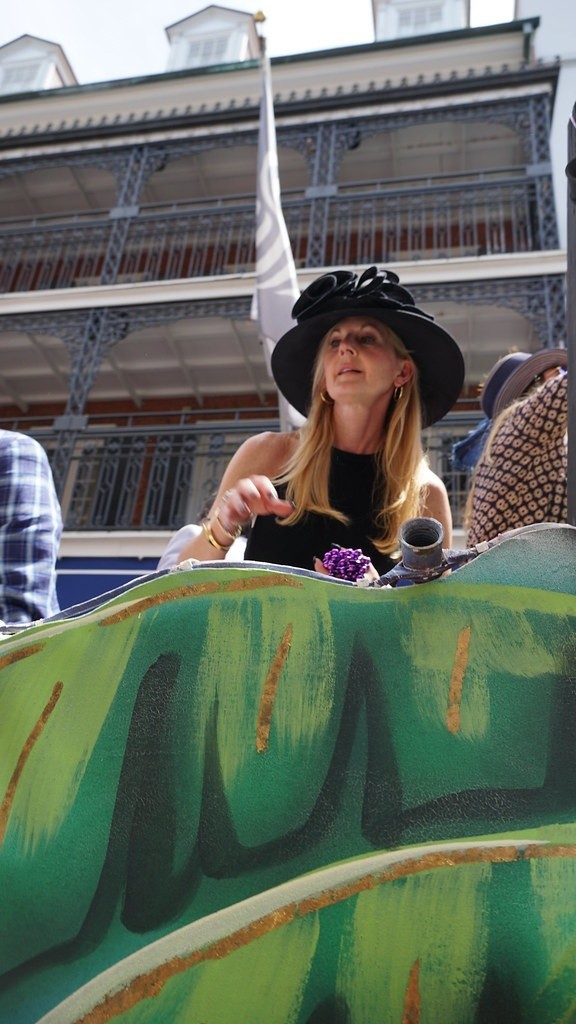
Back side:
[204,508,240,553]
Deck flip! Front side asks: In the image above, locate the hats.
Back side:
[480,349,567,423]
[269,263,463,430]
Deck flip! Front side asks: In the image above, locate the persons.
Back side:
[155,313,453,583]
[466,350,568,550]
[1,428,62,635]
